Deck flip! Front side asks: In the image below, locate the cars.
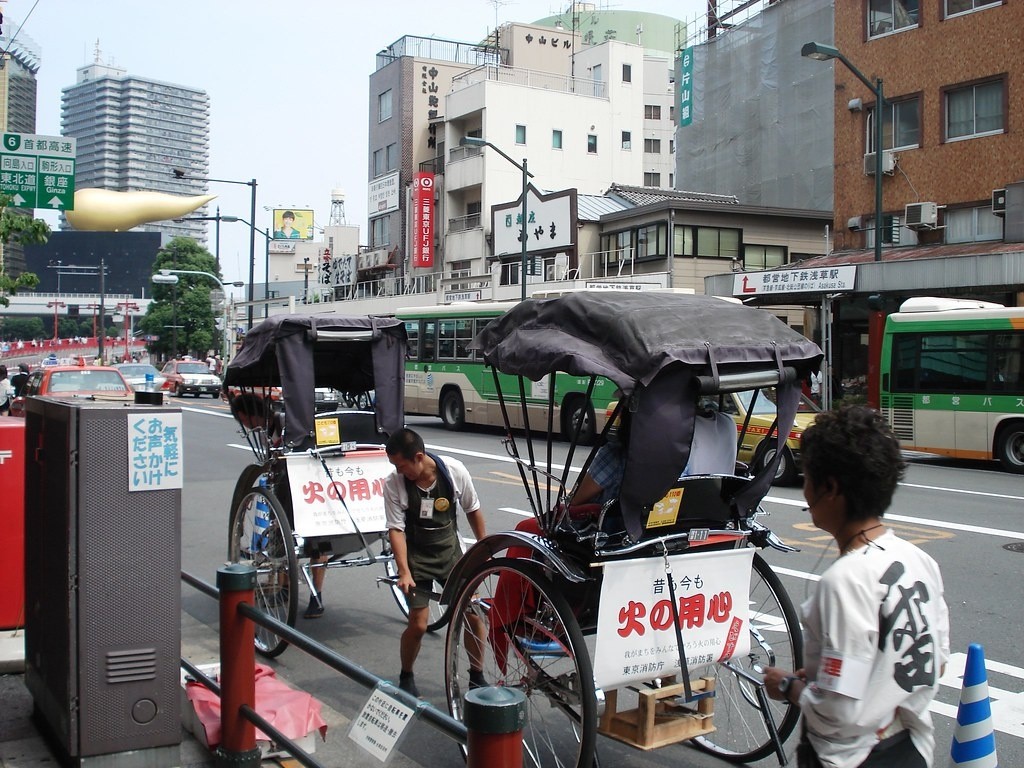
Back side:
[604,382,827,484]
[9,366,137,416]
[97,364,171,403]
[161,356,223,398]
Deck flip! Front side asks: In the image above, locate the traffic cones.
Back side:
[943,641,1000,768]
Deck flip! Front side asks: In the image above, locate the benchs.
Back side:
[495,473,757,633]
[288,408,387,454]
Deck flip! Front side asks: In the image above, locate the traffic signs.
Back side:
[0,153,76,212]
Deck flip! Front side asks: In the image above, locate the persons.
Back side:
[809,364,823,402]
[382,427,497,699]
[273,211,301,238]
[226,393,337,619]
[560,382,634,509]
[0,330,237,419]
[754,408,952,768]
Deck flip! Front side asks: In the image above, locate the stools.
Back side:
[598,675,719,751]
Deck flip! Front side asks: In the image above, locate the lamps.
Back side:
[867,291,885,314]
[292,204,296,209]
[279,204,283,209]
[306,204,309,209]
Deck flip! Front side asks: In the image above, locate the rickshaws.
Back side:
[376,290,806,767]
[221,312,456,659]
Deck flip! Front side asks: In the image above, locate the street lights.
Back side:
[116,303,140,362]
[126,309,140,350]
[460,136,534,303]
[173,204,244,355]
[800,42,885,261]
[46,299,67,345]
[222,215,275,321]
[88,301,100,342]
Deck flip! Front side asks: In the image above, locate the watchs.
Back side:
[778,674,806,707]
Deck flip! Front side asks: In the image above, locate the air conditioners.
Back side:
[547,265,566,281]
[863,150,896,177]
[377,279,398,296]
[359,250,389,268]
[992,189,1006,217]
[905,202,947,232]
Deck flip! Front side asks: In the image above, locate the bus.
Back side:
[392,296,617,447]
[878,293,1023,477]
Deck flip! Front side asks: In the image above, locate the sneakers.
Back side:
[266,584,288,607]
[304,592,324,617]
[399,670,424,699]
[467,669,491,690]
